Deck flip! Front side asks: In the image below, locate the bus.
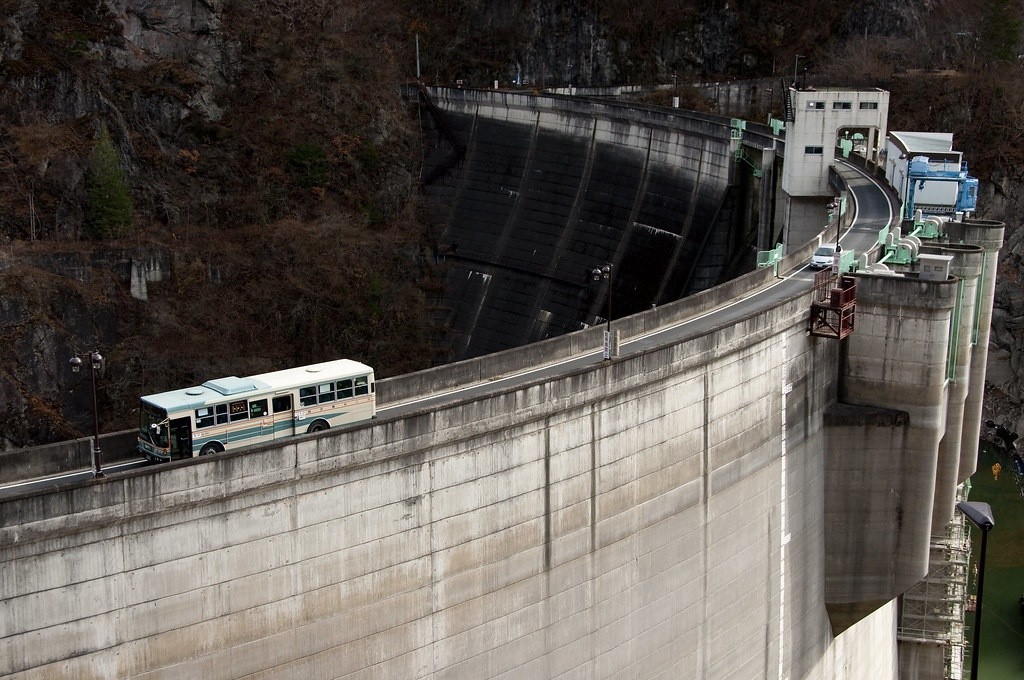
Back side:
[130,359,377,464]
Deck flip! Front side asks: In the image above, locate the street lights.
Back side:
[70,349,107,480]
[567,64,573,95]
[827,201,843,278]
[670,74,677,100]
[592,263,613,361]
[766,87,773,126]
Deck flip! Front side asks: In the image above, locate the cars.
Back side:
[810,242,842,270]
